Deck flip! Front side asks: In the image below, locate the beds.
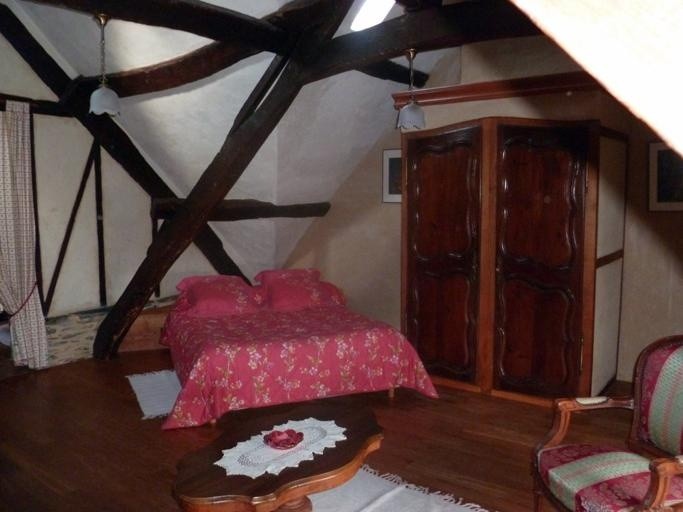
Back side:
[158,281,441,430]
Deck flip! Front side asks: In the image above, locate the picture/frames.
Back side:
[649,142,683,211]
[381,148,405,204]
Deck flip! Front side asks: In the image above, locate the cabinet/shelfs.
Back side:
[399,114,632,413]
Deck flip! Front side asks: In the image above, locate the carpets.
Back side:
[307,463,490,512]
[124,369,181,422]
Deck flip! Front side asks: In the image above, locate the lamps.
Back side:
[87,12,124,118]
[394,47,426,129]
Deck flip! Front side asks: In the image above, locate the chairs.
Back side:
[532,335,683,512]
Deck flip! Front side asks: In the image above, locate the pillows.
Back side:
[176,275,263,317]
[255,267,346,312]
